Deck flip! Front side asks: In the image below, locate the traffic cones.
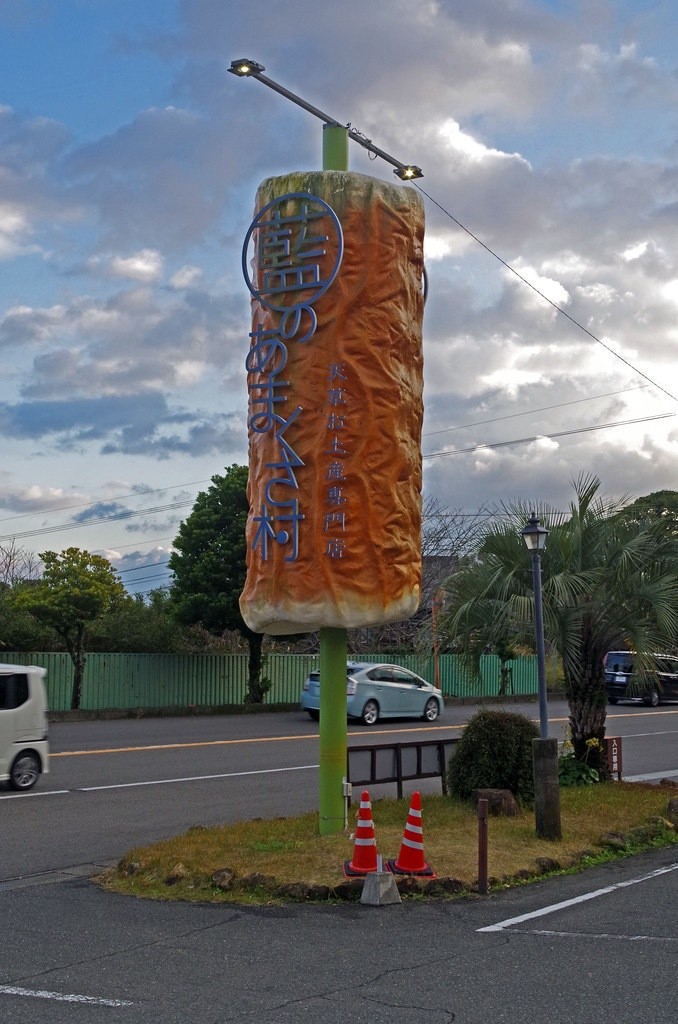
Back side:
[342,789,389,880]
[384,790,438,881]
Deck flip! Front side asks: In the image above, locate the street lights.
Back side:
[225,57,431,836]
[516,511,563,844]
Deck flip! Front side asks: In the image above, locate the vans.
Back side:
[602,651,678,706]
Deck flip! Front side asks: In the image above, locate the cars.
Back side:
[0,663,49,790]
[300,662,447,728]
